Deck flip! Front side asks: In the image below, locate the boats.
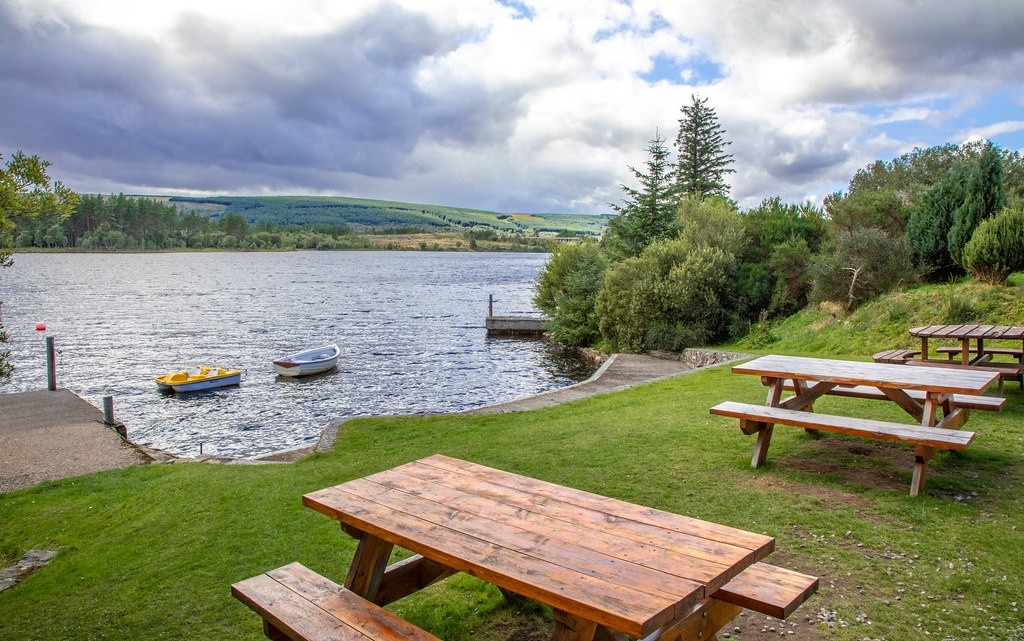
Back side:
[273,344,340,377]
[155,367,241,393]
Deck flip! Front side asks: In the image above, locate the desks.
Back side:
[732,354,999,497]
[909,325,1024,397]
[300,452,775,641]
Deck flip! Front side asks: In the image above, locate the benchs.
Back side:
[906,358,1019,376]
[712,562,820,619]
[711,400,976,451]
[784,379,1007,411]
[871,349,921,364]
[233,561,442,641]
[937,347,1022,353]
